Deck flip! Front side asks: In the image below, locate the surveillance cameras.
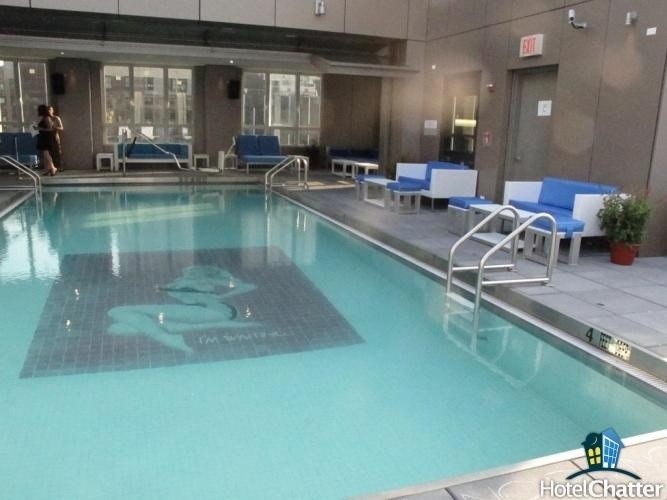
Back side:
[567,8,586,30]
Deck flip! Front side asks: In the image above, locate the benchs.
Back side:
[0,132,42,177]
[236,133,288,174]
[114,142,193,171]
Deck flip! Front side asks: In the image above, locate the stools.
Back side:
[193,153,210,168]
[96,153,114,171]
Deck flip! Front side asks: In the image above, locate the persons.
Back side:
[31,104,58,176]
[103,262,263,357]
[42,191,62,255]
[47,105,65,172]
[53,192,71,251]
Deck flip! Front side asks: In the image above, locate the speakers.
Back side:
[51,74,64,94]
[228,80,238,98]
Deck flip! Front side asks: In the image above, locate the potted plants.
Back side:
[599,193,650,266]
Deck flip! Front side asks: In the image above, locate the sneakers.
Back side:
[44,168,63,176]
